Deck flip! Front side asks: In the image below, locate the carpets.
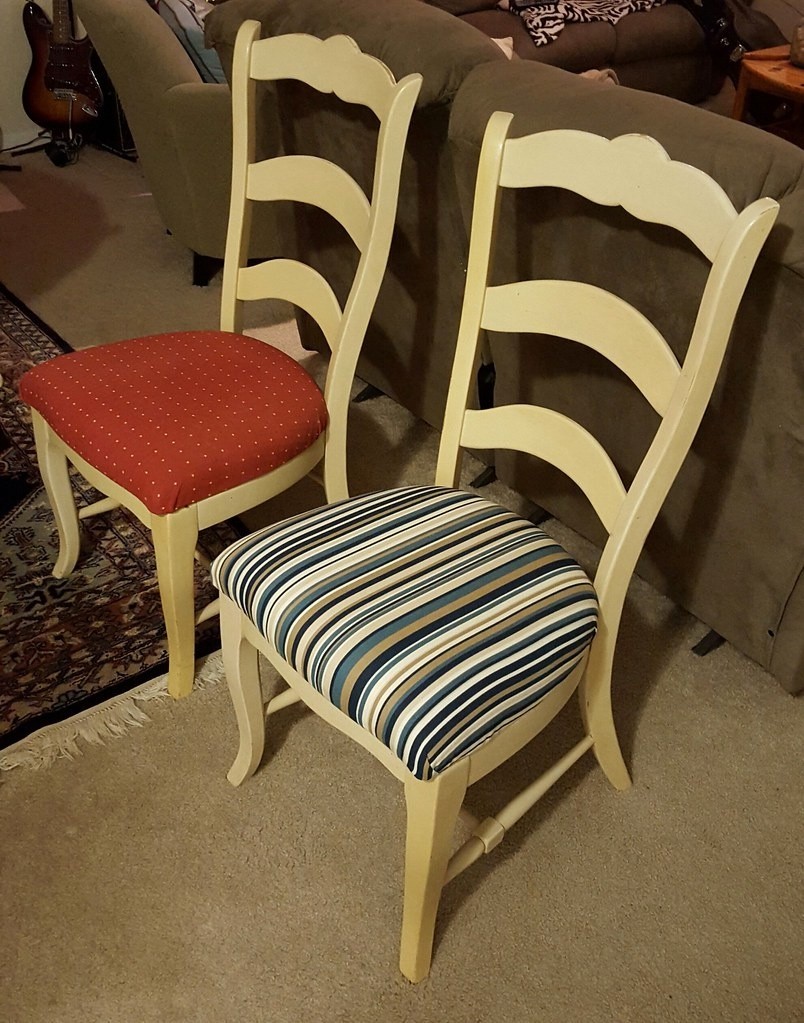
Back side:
[0,283,228,777]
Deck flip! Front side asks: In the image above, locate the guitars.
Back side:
[21,0,106,134]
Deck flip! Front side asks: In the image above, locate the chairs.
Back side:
[20,18,423,699]
[209,108,780,983]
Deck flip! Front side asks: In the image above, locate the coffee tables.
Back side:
[743,49,803,135]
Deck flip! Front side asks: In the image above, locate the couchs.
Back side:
[204,0,511,479]
[68,0,739,282]
[449,61,803,695]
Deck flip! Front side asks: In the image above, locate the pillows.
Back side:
[153,0,227,83]
